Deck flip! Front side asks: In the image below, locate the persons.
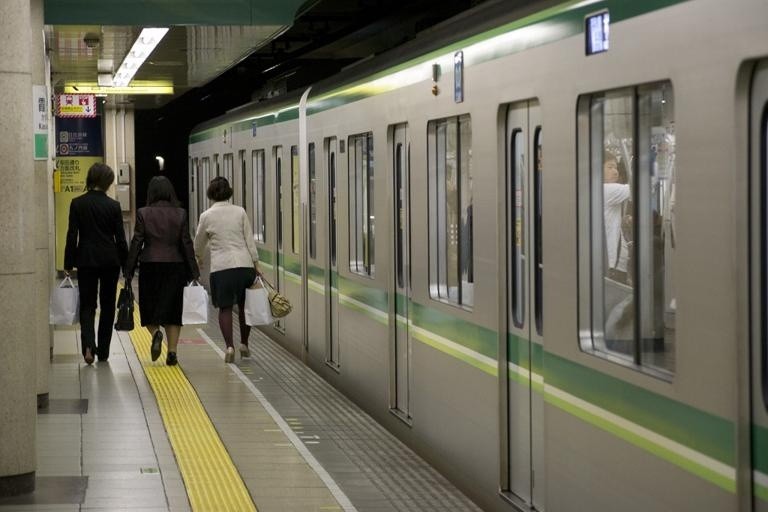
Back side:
[123,175,200,366]
[63,162,128,365]
[193,177,262,363]
[601,150,632,283]
[446,165,457,227]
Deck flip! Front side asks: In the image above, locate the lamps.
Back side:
[113,28,170,87]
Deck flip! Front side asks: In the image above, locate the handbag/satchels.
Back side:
[49,287,80,325]
[182,287,209,325]
[117,288,134,311]
[244,288,280,326]
[115,304,134,330]
[268,291,293,317]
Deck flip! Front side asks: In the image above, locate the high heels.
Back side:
[225,347,234,363]
[151,331,162,361]
[239,344,250,359]
[85,343,94,364]
[166,352,176,366]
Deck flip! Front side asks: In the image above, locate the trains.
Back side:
[188,0,767,511]
